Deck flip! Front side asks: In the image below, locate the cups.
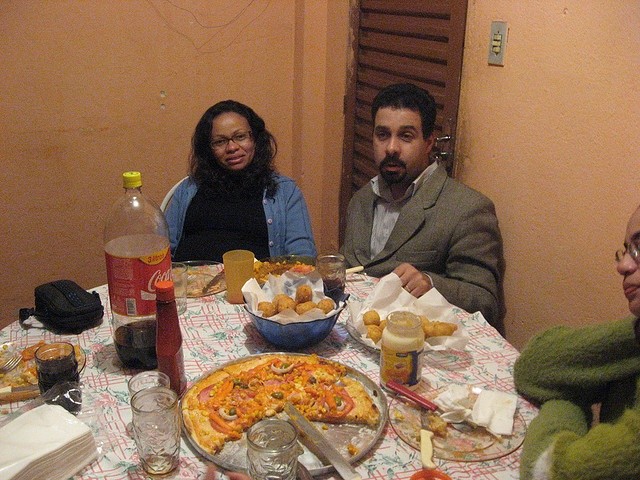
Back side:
[209,130,253,150]
[615,235,640,262]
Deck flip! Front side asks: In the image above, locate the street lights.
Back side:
[18,278,104,336]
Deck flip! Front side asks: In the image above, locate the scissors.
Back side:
[386,380,478,435]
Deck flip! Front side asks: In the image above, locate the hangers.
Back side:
[386,380,479,435]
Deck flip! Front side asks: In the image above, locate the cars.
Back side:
[33,342,82,416]
[127,370,171,397]
[171,262,188,316]
[315,251,347,300]
[223,250,255,305]
[246,418,304,480]
[131,386,180,475]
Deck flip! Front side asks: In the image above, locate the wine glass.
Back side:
[406,285,411,293]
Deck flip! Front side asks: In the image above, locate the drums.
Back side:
[419,407,436,469]
[284,403,362,480]
[0,384,39,394]
[203,269,225,293]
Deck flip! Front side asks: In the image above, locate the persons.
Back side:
[340,82,504,327]
[154,100,317,264]
[513,202,639,480]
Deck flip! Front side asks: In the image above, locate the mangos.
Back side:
[183,352,383,457]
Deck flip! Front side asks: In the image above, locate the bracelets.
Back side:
[242,281,351,351]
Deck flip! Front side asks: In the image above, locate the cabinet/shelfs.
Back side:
[154,281,188,400]
[378,310,425,393]
[103,169,176,372]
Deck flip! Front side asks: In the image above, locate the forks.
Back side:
[0,353,24,374]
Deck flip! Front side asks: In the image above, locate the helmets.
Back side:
[252,255,321,289]
[388,382,527,463]
[177,351,388,476]
[345,315,434,355]
[0,338,87,409]
[171,260,227,298]
